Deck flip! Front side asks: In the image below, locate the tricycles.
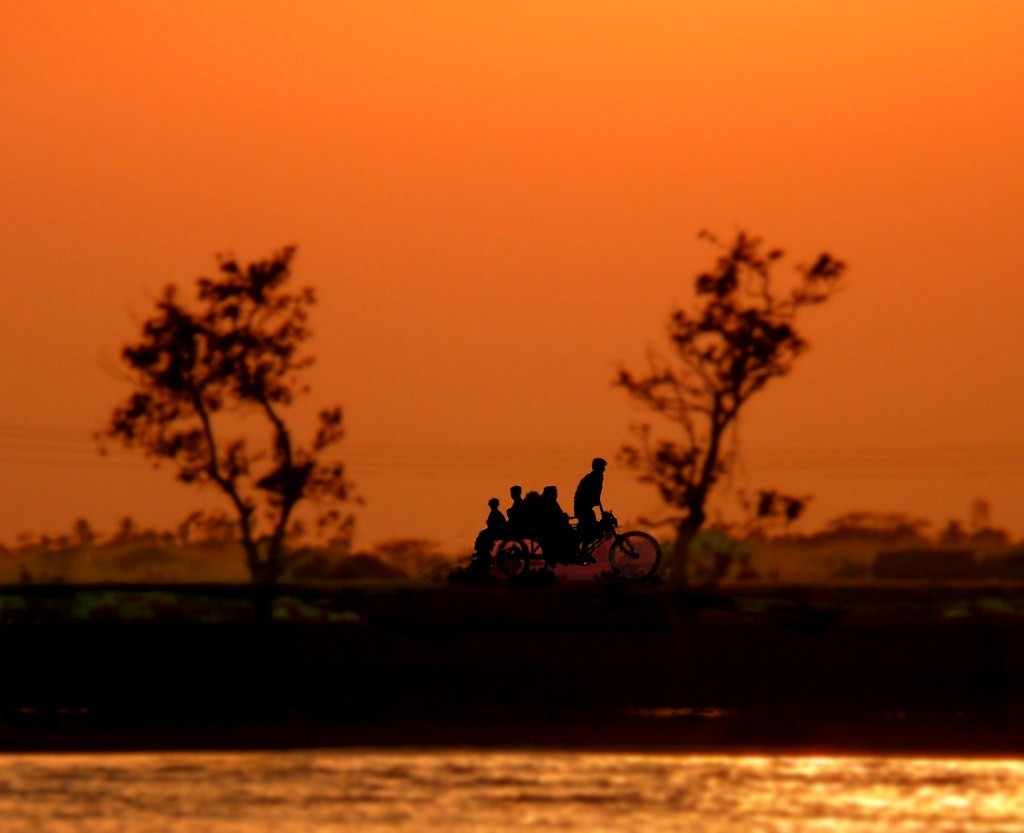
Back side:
[493,507,662,582]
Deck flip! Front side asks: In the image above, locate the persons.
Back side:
[474,458,606,564]
